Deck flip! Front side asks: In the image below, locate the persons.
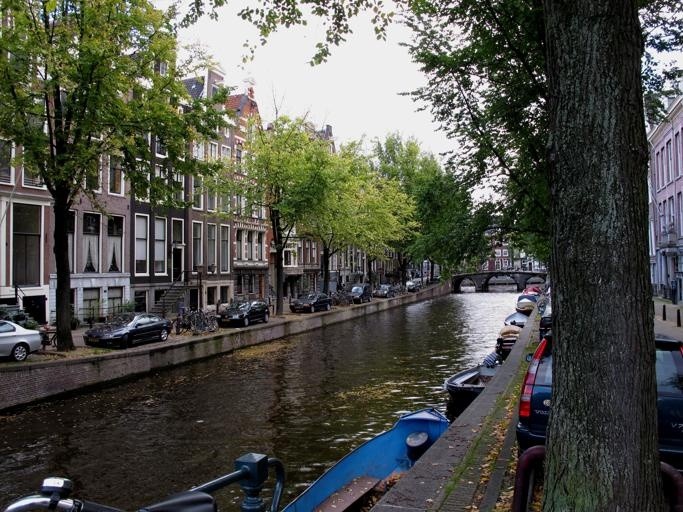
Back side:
[483,350,502,368]
[216,300,220,313]
[529,259,532,271]
[522,261,526,271]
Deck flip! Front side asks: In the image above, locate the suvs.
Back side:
[515,327,683,475]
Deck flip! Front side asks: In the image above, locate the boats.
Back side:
[281,406,451,512]
[444,284,542,400]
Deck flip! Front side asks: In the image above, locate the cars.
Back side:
[217,299,270,325]
[0,319,43,361]
[82,311,174,349]
[289,291,333,314]
[351,282,395,304]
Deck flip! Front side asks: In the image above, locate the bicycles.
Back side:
[170,309,217,334]
